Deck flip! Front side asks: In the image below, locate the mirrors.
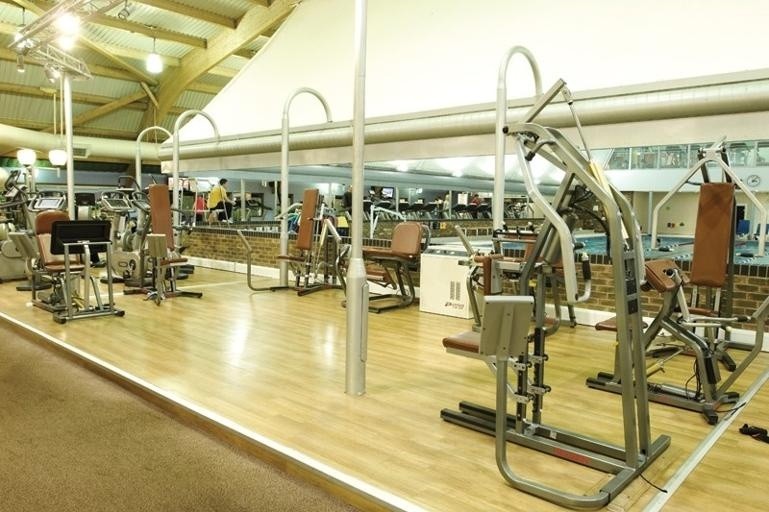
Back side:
[177,140,769,267]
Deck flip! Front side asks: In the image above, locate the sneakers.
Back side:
[221,219,234,224]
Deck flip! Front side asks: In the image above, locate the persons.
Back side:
[207,178,236,224]
[342,184,352,208]
[194,195,206,220]
[471,194,480,205]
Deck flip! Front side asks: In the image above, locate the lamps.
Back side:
[16,54,24,72]
[16,149,38,168]
[47,150,69,179]
[117,0,135,19]
[146,36,163,74]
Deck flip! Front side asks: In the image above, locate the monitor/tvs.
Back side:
[34,197,62,210]
[382,186,394,198]
[5,184,25,198]
[104,198,131,210]
